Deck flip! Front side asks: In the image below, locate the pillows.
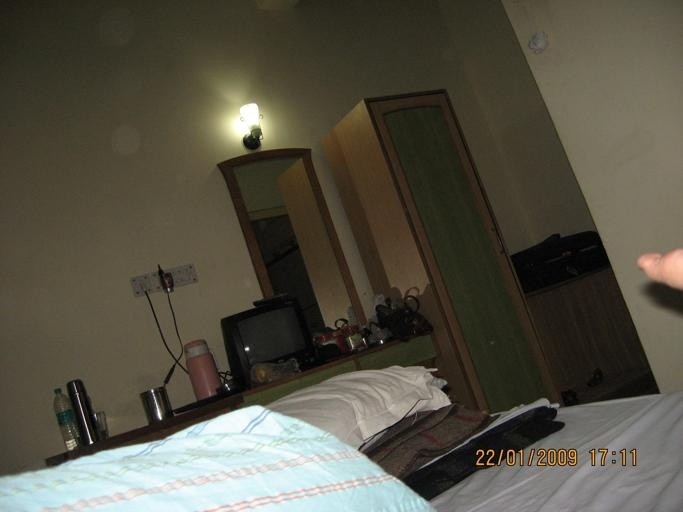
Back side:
[254,366,453,452]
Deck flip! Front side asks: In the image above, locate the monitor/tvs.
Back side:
[221,294,313,390]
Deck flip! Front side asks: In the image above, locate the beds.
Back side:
[0,391,683,510]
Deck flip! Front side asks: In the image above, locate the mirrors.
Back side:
[216,148,369,343]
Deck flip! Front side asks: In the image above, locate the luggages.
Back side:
[509,231,610,294]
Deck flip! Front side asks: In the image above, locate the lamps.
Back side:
[237,102,264,150]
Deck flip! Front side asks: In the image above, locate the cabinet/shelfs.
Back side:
[521,265,651,407]
[320,87,561,415]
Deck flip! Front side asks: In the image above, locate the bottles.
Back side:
[53,377,99,453]
[183,339,224,402]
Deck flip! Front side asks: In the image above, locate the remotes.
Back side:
[253,293,288,307]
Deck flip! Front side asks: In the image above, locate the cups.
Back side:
[92,409,107,441]
[140,386,174,424]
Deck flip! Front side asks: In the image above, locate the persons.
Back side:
[634,247,682,290]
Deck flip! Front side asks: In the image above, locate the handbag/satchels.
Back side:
[374,304,433,336]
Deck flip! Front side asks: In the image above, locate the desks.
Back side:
[44,330,442,466]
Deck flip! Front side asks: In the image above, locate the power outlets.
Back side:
[129,262,199,298]
